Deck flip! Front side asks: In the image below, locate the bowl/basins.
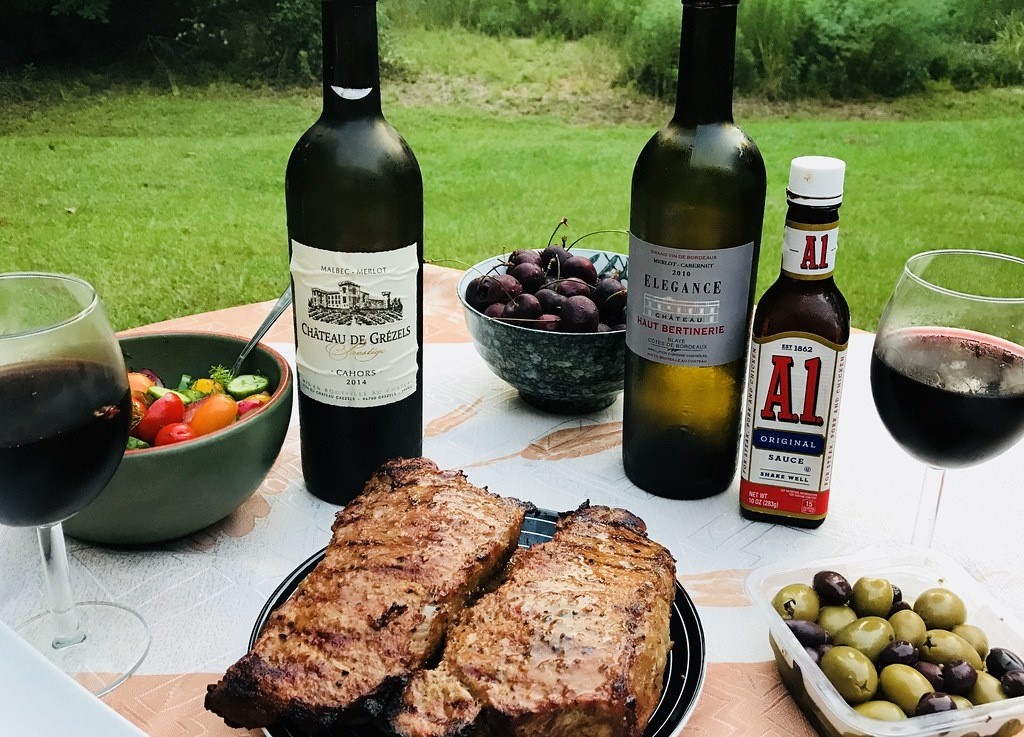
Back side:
[457,248,629,415]
[743,545,1024,737]
[62,331,293,545]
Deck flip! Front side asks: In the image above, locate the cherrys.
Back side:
[423,216,631,334]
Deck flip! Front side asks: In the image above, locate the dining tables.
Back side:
[36,260,1024,737]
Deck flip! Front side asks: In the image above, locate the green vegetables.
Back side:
[207,364,236,391]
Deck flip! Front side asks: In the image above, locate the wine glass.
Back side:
[870,249,1023,603]
[1,271,153,699]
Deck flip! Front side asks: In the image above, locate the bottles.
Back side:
[621,1,767,501]
[739,155,850,530]
[283,0,424,506]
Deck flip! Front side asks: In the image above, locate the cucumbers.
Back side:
[146,373,269,406]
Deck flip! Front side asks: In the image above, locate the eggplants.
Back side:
[129,392,238,448]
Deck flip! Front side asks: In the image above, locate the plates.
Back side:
[247,508,707,737]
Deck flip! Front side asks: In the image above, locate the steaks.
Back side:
[202,457,678,737]
